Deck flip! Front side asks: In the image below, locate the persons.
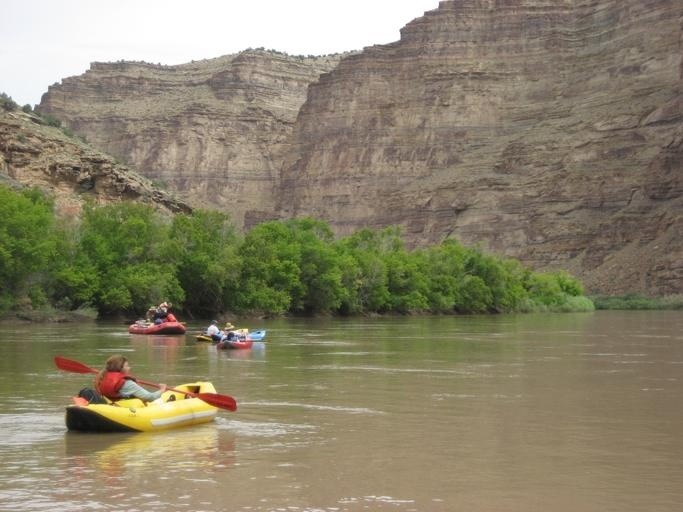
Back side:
[95,355,167,402]
[145,301,176,325]
[207,319,246,342]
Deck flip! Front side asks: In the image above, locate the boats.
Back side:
[243,329,267,341]
[62,376,219,436]
[195,327,250,342]
[127,320,187,335]
[215,339,254,350]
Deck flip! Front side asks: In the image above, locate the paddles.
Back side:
[56,358,236,411]
[123,321,186,325]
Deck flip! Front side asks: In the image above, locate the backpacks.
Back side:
[78,387,108,405]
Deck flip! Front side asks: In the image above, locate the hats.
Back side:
[224,322,234,330]
[211,319,218,324]
[149,307,156,311]
